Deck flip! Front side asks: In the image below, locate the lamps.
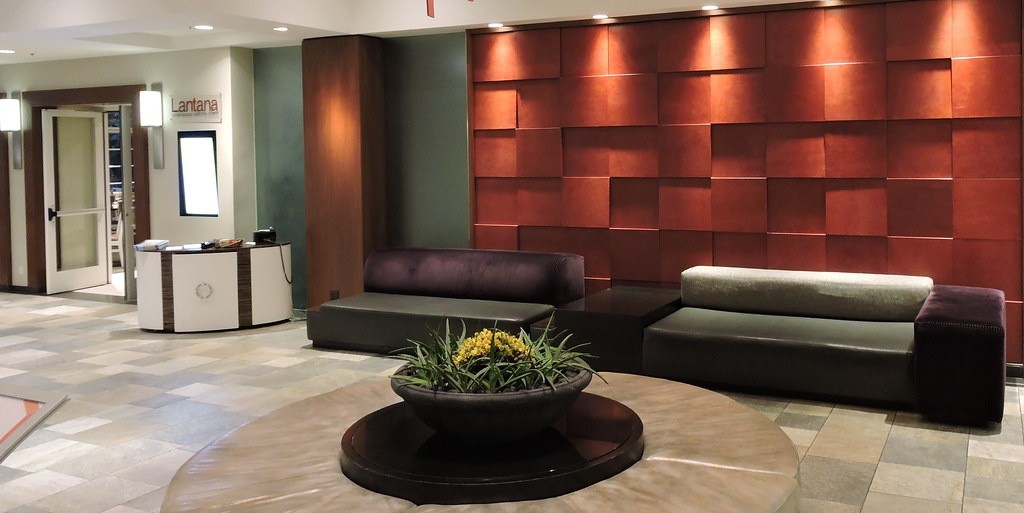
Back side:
[139,91,162,127]
[0,99,21,132]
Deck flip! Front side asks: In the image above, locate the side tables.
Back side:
[551,285,684,379]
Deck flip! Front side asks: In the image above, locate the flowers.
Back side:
[391,315,609,395]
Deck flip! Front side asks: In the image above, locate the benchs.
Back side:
[641,265,1007,426]
[304,245,586,357]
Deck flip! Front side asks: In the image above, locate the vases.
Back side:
[387,356,609,443]
